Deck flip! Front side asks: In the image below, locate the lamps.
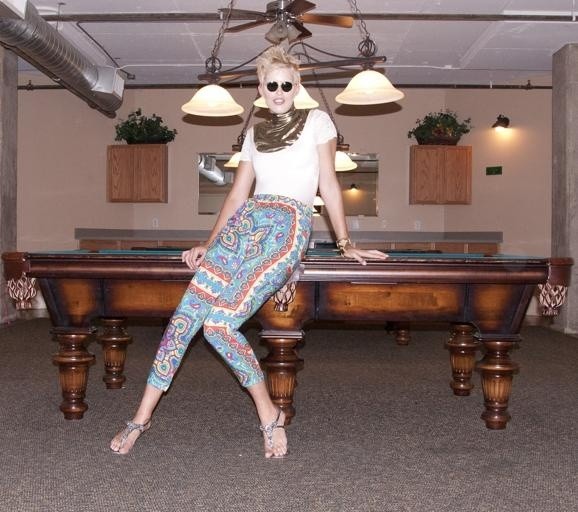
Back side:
[492,114,510,129]
[349,184,358,191]
[223,67,358,172]
[312,152,358,207]
[180,0,406,119]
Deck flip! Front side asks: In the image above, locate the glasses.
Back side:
[267,81,292,91]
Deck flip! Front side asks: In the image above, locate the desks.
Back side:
[2,248,573,432]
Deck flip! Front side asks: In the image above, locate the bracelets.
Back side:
[335,237,353,255]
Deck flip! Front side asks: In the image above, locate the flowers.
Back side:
[406,105,470,140]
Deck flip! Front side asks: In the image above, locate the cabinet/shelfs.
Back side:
[408,145,473,206]
[77,238,499,256]
[106,144,169,204]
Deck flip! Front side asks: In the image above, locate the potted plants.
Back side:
[113,108,177,144]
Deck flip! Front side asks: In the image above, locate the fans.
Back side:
[213,1,354,44]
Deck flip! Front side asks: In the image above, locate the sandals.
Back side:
[109,421,150,454]
[260,408,289,459]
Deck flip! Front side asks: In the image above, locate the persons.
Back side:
[107,44,391,458]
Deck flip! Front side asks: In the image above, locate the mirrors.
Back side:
[196,151,379,217]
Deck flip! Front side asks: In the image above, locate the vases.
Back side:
[416,137,460,145]
